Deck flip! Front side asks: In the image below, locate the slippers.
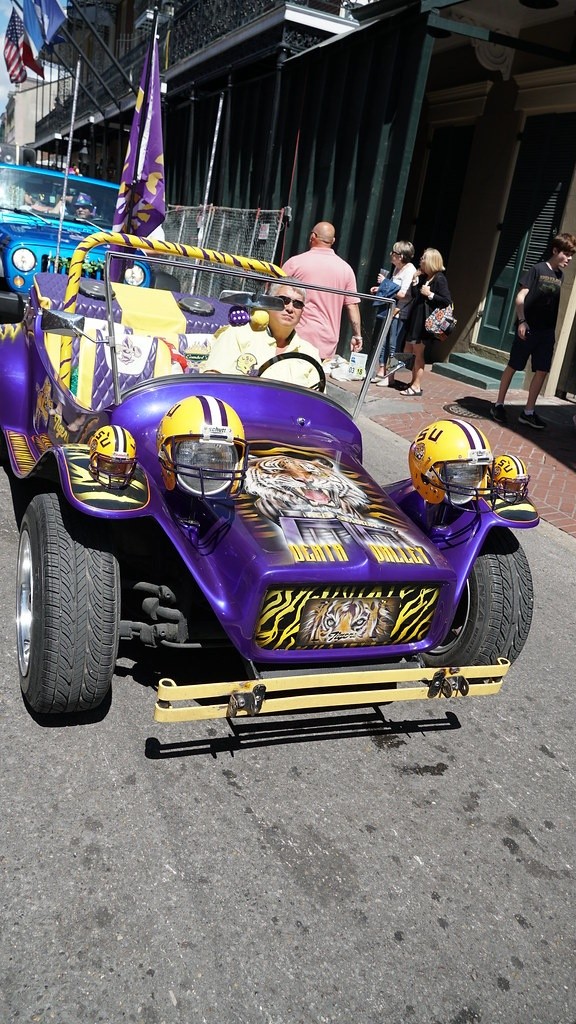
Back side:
[400,387,422,396]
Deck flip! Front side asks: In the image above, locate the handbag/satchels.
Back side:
[425,302,457,342]
[348,351,368,380]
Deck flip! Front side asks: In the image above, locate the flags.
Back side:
[3,0,65,83]
[109,37,166,282]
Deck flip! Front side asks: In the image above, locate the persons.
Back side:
[395,248,451,396]
[25,168,78,214]
[371,241,417,385]
[73,193,95,219]
[491,233,576,427]
[207,277,326,395]
[282,222,362,362]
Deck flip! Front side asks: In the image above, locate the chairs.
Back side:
[72,328,172,411]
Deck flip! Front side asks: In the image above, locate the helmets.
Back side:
[409,419,498,513]
[88,425,137,490]
[158,395,249,500]
[488,455,531,504]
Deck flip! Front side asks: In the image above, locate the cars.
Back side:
[1,233,539,727]
[0,162,151,322]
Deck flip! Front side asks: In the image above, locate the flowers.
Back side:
[50,257,106,273]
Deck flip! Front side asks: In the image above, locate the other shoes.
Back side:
[371,375,383,382]
[376,379,396,386]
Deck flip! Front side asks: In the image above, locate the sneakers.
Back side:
[518,409,547,428]
[490,404,506,422]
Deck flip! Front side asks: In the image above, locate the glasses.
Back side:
[392,251,401,255]
[75,205,89,209]
[279,295,305,309]
